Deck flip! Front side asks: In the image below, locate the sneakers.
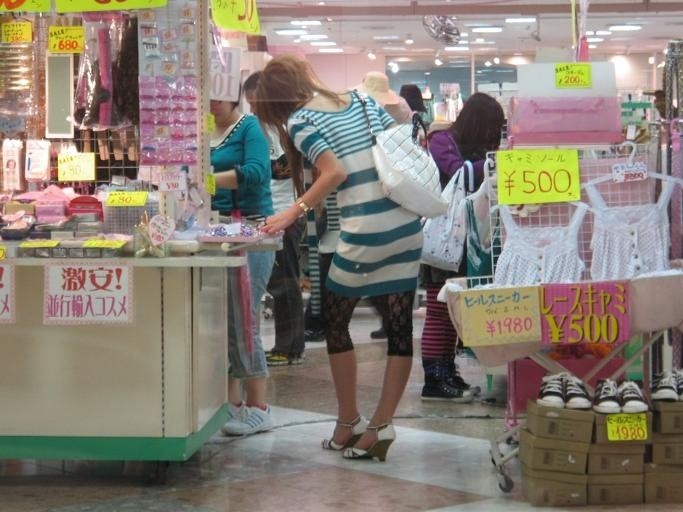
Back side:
[221,406,273,435]
[594,379,621,413]
[618,379,648,412]
[537,372,565,408]
[675,370,683,402]
[564,374,592,409]
[303,328,328,341]
[262,349,304,366]
[226,400,245,419]
[651,373,679,401]
[371,326,388,339]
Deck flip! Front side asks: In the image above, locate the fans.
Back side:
[421,14,461,49]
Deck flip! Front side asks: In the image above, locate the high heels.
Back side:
[321,415,369,450]
[342,422,396,461]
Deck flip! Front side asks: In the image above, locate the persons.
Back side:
[421,93,506,403]
[243,68,309,367]
[258,52,424,463]
[208,74,277,438]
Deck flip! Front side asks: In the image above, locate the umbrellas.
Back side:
[231,165,256,370]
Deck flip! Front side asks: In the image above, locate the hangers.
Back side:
[577,141,683,192]
[489,180,596,221]
[458,159,494,210]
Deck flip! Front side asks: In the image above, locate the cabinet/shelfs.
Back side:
[1,234,276,461]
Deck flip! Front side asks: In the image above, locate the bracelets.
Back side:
[295,197,310,214]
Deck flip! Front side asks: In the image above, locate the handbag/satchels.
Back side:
[420,167,469,272]
[353,86,448,219]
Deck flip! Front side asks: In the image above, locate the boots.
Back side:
[421,357,473,403]
[442,354,481,395]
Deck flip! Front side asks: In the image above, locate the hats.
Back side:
[399,85,427,112]
[351,71,398,105]
[383,97,417,126]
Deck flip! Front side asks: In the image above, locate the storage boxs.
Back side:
[520,462,587,506]
[525,400,590,441]
[518,428,586,473]
[653,401,683,433]
[586,472,644,505]
[645,463,683,506]
[651,434,683,465]
[588,445,645,473]
[595,412,653,443]
